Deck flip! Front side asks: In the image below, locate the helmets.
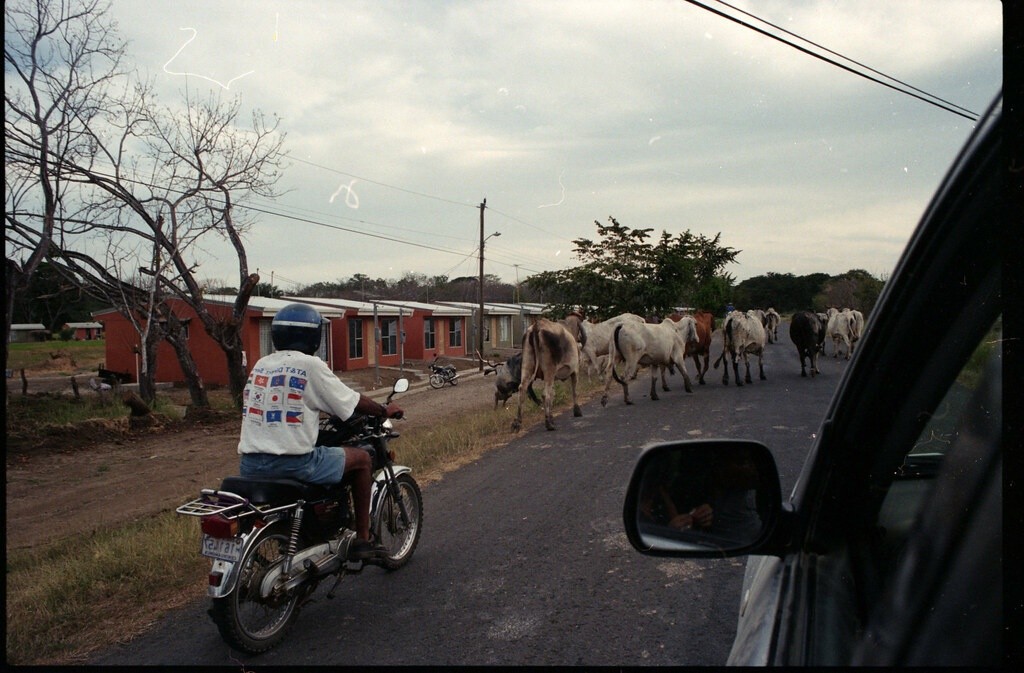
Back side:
[271,304,331,355]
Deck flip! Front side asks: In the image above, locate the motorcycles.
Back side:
[426,352,462,389]
[176,378,423,656]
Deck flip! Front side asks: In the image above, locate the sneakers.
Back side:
[351,533,391,559]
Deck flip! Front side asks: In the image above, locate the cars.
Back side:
[622,79,1002,668]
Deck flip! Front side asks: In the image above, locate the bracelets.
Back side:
[380,403,388,417]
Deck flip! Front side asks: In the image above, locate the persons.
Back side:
[726,302,735,315]
[237,303,405,560]
[638,447,715,532]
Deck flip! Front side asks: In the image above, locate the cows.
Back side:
[483,307,865,410]
[509,311,588,434]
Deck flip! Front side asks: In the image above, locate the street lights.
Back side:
[479,232,503,371]
